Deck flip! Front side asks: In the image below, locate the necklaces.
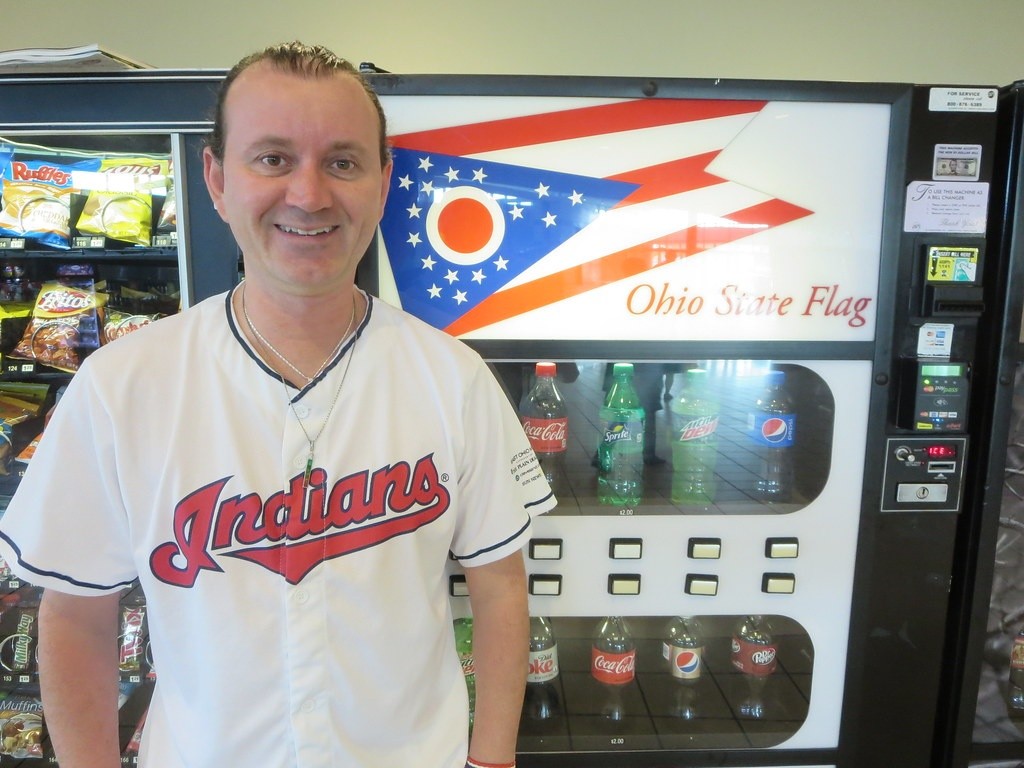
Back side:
[242,285,357,489]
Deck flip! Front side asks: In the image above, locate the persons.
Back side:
[0,44,558,768]
[584,363,698,474]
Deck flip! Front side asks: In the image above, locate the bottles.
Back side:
[517,362,569,495]
[667,615,703,734]
[669,369,721,503]
[745,370,798,502]
[522,617,563,736]
[596,363,647,507]
[455,619,474,736]
[730,614,778,729]
[590,616,636,736]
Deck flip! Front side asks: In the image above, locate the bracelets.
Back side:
[464,755,516,768]
